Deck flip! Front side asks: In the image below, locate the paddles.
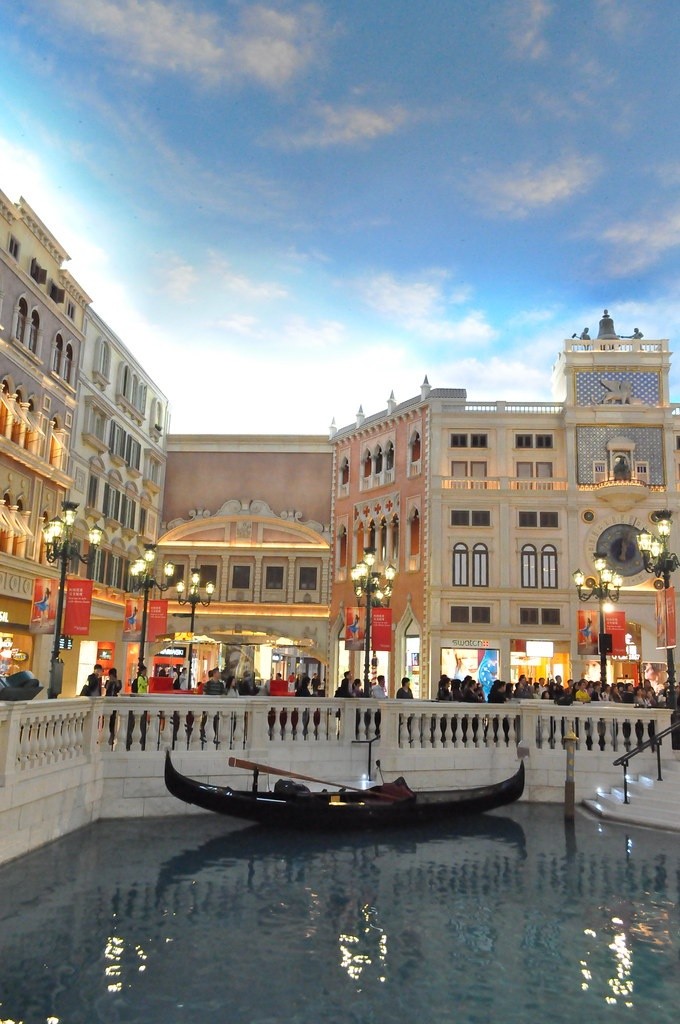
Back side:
[227,757,407,804]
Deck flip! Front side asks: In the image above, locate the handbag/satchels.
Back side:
[80,684,91,696]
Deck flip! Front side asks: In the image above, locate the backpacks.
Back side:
[173,675,185,690]
[131,676,148,694]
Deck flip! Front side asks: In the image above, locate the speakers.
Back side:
[599,634,612,653]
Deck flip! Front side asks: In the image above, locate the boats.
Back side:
[164,748,525,832]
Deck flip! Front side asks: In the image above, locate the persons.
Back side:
[80,664,103,696]
[454,648,480,684]
[104,668,123,697]
[579,618,592,648]
[138,665,149,693]
[157,664,680,750]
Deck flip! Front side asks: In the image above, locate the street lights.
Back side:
[129,544,175,677]
[349,545,397,696]
[177,570,215,692]
[572,552,623,701]
[43,500,103,699]
[634,507,680,709]
